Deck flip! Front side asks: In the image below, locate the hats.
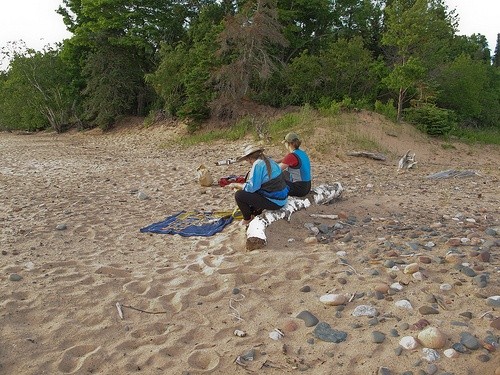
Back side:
[281,133,299,144]
[238,144,264,162]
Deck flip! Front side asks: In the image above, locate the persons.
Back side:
[228,145,289,224]
[279,132,312,197]
